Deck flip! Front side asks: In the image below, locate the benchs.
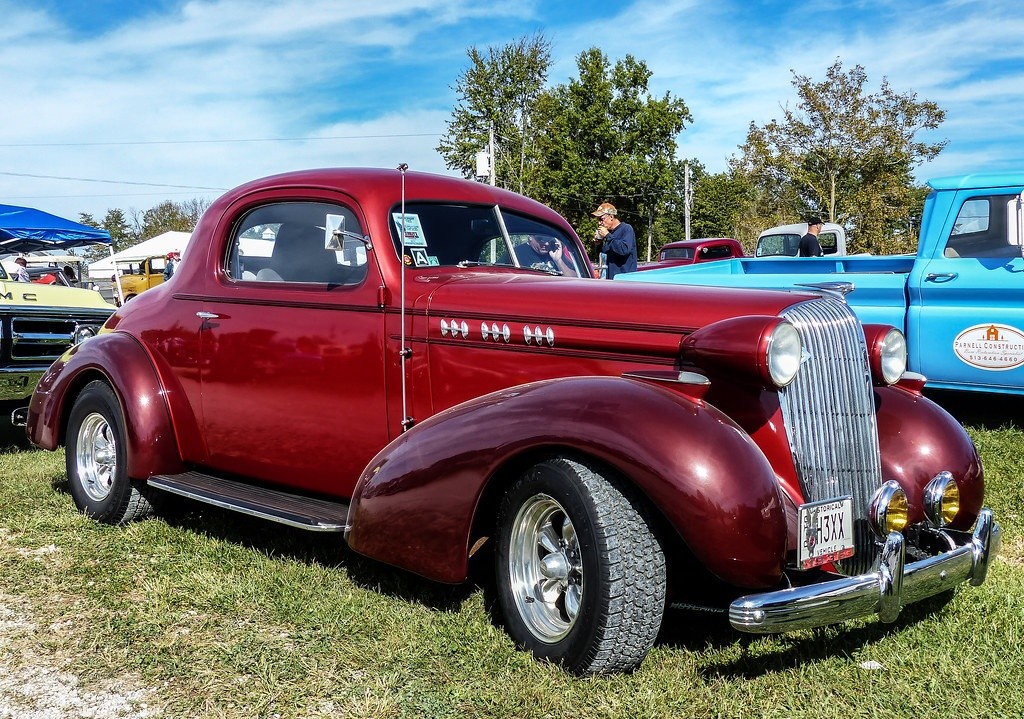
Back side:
[945,238,1004,257]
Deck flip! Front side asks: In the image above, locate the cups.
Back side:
[596,230,603,239]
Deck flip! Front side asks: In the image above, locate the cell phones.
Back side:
[550,239,558,251]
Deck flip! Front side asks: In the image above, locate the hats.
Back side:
[808,217,825,226]
[165,253,173,260]
[591,203,617,217]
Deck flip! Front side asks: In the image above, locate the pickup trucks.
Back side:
[612,166,1024,428]
[753,222,873,259]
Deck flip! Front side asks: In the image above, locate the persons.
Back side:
[591,203,637,281]
[494,234,577,276]
[256,220,368,284]
[799,216,825,257]
[17,249,180,281]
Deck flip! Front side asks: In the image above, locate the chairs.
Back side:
[256,222,338,284]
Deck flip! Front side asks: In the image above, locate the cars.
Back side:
[13,162,1004,677]
[0,249,182,438]
[636,236,754,272]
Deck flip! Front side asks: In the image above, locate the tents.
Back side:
[0,204,124,307]
[88,231,368,289]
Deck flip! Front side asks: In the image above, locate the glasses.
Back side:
[536,237,554,244]
[598,215,610,221]
